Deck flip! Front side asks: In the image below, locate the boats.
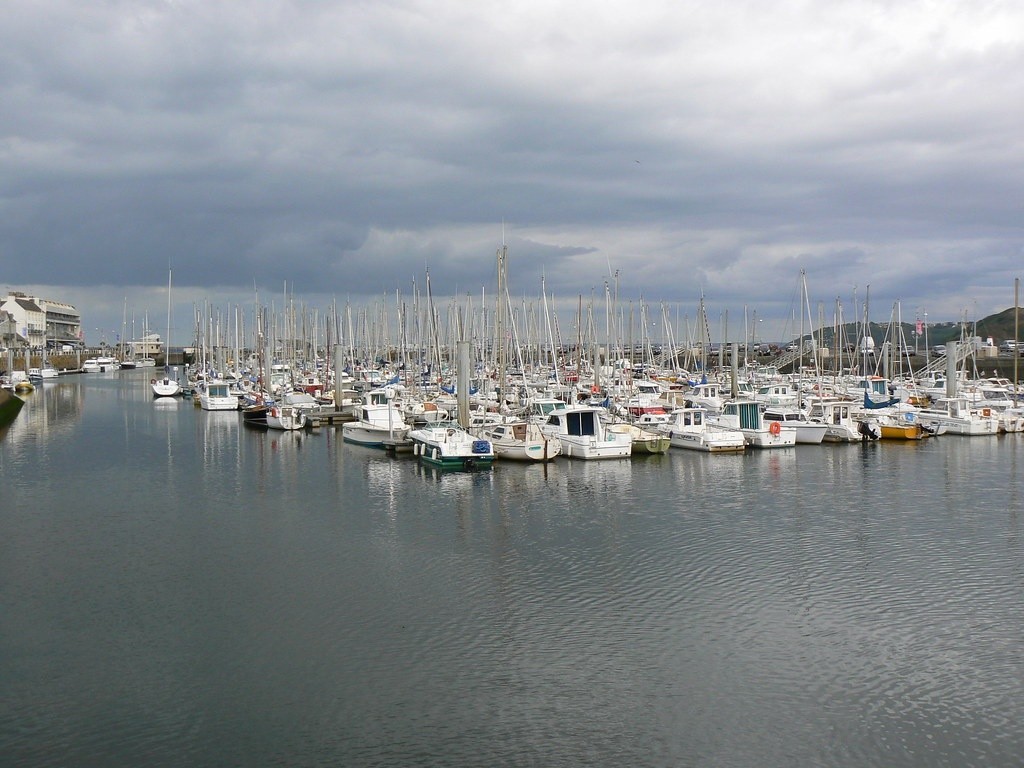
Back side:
[81,327,122,373]
[1,370,30,388]
[41,359,60,378]
[28,367,43,384]
[15,375,34,390]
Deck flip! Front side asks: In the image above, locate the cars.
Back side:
[542,342,779,356]
[859,346,875,357]
[930,345,946,357]
[902,345,916,356]
[999,340,1019,352]
[785,342,799,352]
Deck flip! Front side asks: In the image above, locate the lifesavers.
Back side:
[769,422,781,435]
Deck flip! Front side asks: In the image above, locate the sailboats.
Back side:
[150,268,184,396]
[121,295,157,368]
[187,241,1024,475]
